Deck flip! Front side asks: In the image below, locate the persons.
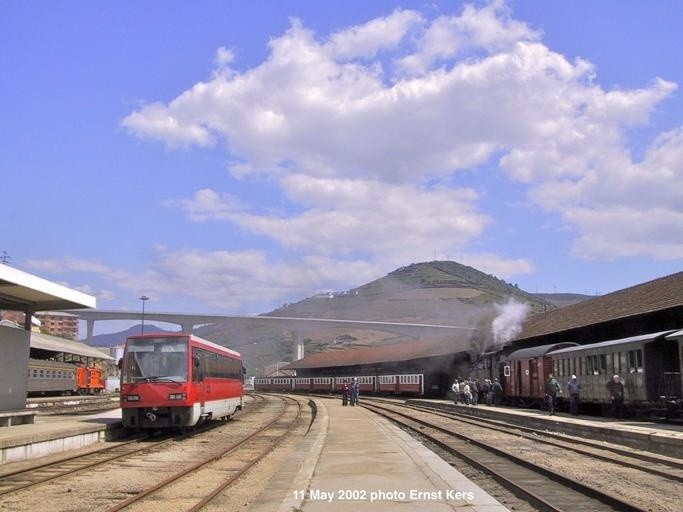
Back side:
[607,374,625,419]
[544,374,563,416]
[451,375,503,407]
[565,374,583,416]
[342,377,359,407]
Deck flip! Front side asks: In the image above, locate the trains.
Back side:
[27,359,105,396]
[118,332,246,432]
[254,328,683,423]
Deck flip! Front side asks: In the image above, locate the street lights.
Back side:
[140,295,149,333]
